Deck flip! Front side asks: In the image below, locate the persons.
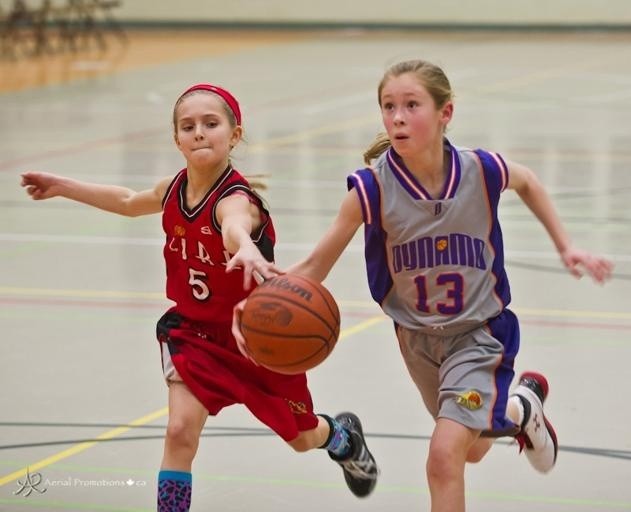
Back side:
[235,61,611,512]
[22,84,378,512]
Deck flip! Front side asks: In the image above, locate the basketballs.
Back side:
[240,273,341,376]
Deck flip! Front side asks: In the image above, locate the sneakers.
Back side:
[513,373,558,472]
[330,413,377,498]
